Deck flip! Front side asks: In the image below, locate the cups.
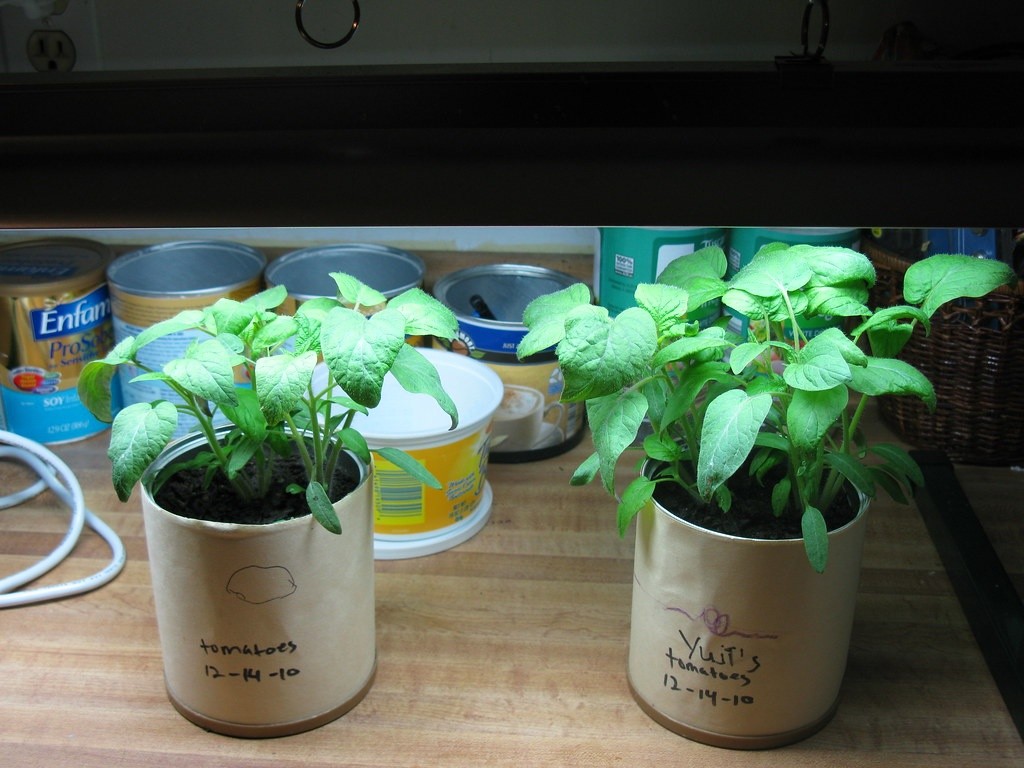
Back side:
[490,384,565,452]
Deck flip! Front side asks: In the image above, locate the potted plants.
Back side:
[518,242,1017,750]
[76,271,458,738]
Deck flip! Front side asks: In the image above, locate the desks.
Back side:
[0,398,1024,768]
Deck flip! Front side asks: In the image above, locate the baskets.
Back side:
[859,227,1024,467]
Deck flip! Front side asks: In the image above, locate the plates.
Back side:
[534,423,564,450]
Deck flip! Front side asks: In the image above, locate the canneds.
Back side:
[0,226,863,462]
